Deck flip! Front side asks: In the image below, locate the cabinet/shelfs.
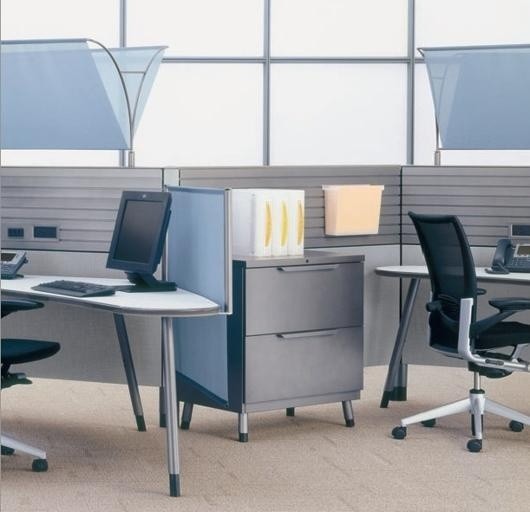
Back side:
[180,248,366,443]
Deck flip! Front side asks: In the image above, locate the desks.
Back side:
[0,278,220,498]
[374,262,529,408]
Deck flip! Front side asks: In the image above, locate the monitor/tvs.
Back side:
[106,190,177,291]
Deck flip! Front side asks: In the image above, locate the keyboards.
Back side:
[30,279,116,298]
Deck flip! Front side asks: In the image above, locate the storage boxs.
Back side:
[226,188,305,259]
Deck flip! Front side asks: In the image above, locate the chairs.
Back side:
[391,210,530,453]
[0,299,61,472]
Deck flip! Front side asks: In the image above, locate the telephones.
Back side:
[491,238,530,273]
